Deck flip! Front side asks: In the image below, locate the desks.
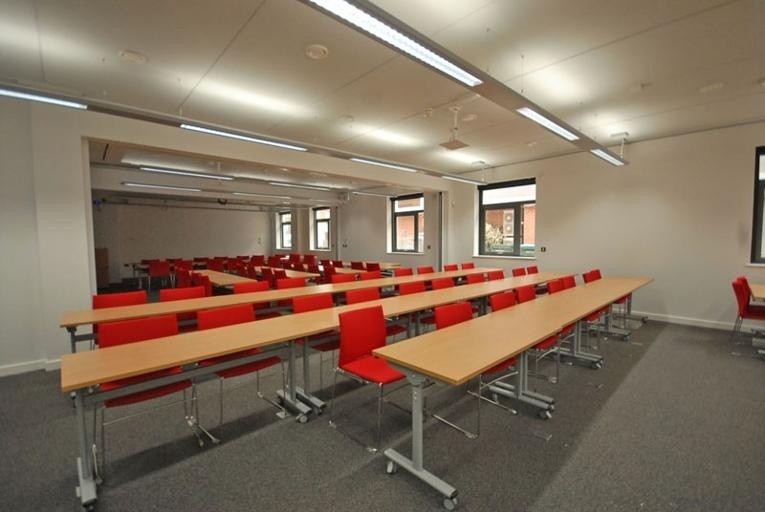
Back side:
[749,284,765,357]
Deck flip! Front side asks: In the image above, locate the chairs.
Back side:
[730,276,765,345]
